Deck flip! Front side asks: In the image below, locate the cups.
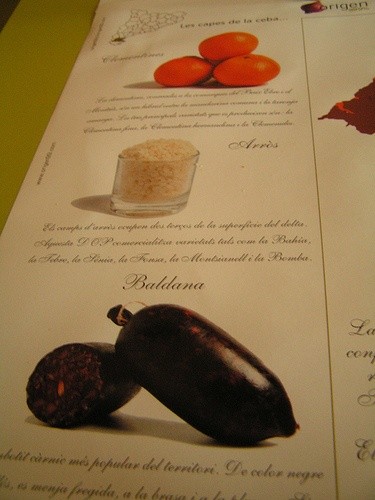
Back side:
[111,151,202,216]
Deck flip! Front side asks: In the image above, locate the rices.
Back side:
[115,137,198,201]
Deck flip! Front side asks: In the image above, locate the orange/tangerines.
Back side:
[154,31,280,87]
